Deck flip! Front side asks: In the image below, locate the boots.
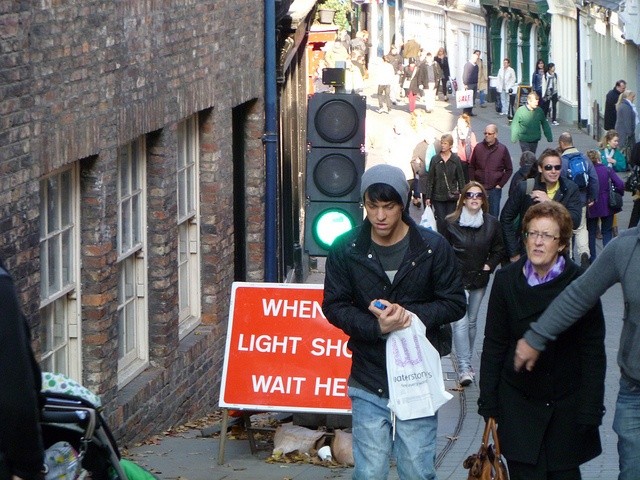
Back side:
[612,226,619,236]
[595,224,603,239]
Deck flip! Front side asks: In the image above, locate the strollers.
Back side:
[39,389,155,480]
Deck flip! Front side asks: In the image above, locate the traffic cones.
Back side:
[513,86,533,110]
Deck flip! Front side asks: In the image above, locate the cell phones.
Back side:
[374,303,387,309]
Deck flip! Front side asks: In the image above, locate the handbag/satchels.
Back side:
[607,166,623,215]
[456,88,473,109]
[448,82,452,94]
[420,204,438,232]
[620,135,633,164]
[496,92,502,112]
[440,162,460,200]
[402,67,418,89]
[39,406,96,480]
[463,417,510,480]
[384,309,456,441]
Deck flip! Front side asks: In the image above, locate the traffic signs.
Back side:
[217,282,354,465]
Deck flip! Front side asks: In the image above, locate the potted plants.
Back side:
[316,0,351,30]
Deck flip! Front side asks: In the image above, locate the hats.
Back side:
[361,164,409,206]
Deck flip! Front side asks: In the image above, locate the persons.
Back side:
[322,164,466,480]
[451,113,477,184]
[463,54,479,116]
[510,91,553,154]
[400,57,419,113]
[467,124,513,219]
[595,129,627,239]
[434,47,450,100]
[586,149,624,264]
[387,44,402,105]
[322,29,372,92]
[532,59,547,109]
[425,134,466,247]
[542,63,560,126]
[477,200,607,480]
[417,52,444,113]
[514,221,640,480]
[500,149,582,264]
[496,58,516,115]
[376,55,394,114]
[473,50,488,108]
[559,132,600,273]
[411,127,440,209]
[615,90,639,153]
[604,80,626,130]
[438,181,505,386]
[625,143,640,228]
[509,151,536,197]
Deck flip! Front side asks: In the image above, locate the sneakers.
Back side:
[459,372,473,387]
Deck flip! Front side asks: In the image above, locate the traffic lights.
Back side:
[303,94,366,257]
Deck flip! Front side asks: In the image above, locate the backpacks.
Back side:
[562,153,589,189]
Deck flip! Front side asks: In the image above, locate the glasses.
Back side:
[544,164,561,170]
[524,231,561,241]
[483,132,496,135]
[465,192,484,200]
